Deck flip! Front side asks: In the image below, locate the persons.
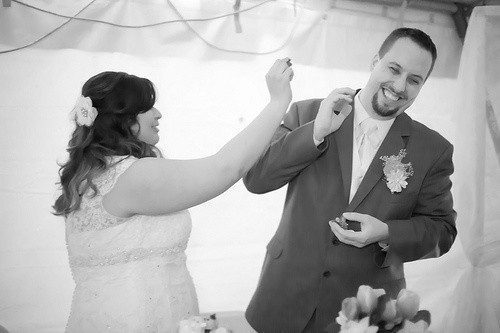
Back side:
[51,58,295,333]
[243,26,459,333]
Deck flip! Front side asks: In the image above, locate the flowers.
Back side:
[335,284,432,333]
[380,147,416,194]
[65,91,99,127]
